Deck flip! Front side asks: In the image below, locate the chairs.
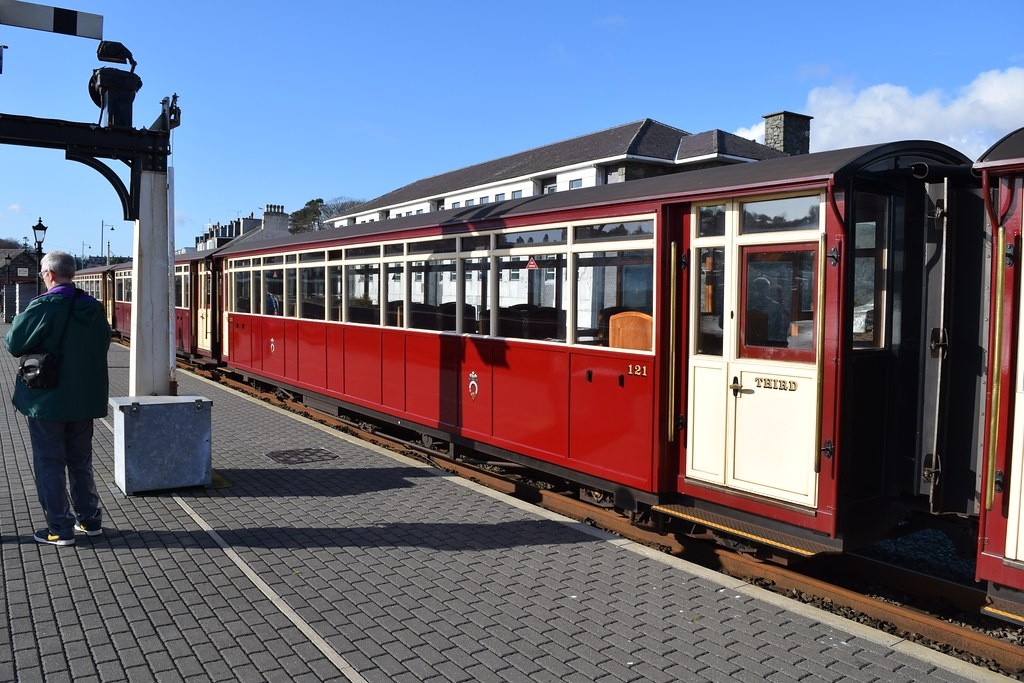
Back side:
[599,306,652,353]
[266,296,568,343]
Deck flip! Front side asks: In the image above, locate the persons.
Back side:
[5,251,111,546]
[266,292,279,315]
[747,277,790,347]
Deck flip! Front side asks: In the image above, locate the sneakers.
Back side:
[73,519,103,536]
[34,528,75,545]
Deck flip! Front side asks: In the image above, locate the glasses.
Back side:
[38,270,55,278]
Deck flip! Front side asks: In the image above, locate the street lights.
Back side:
[32,216,48,297]
[82,240,91,269]
[5,254,12,285]
[101,220,115,266]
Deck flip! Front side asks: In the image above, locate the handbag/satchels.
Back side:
[18,353,58,388]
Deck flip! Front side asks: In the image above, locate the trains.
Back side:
[70,128,1023,625]
